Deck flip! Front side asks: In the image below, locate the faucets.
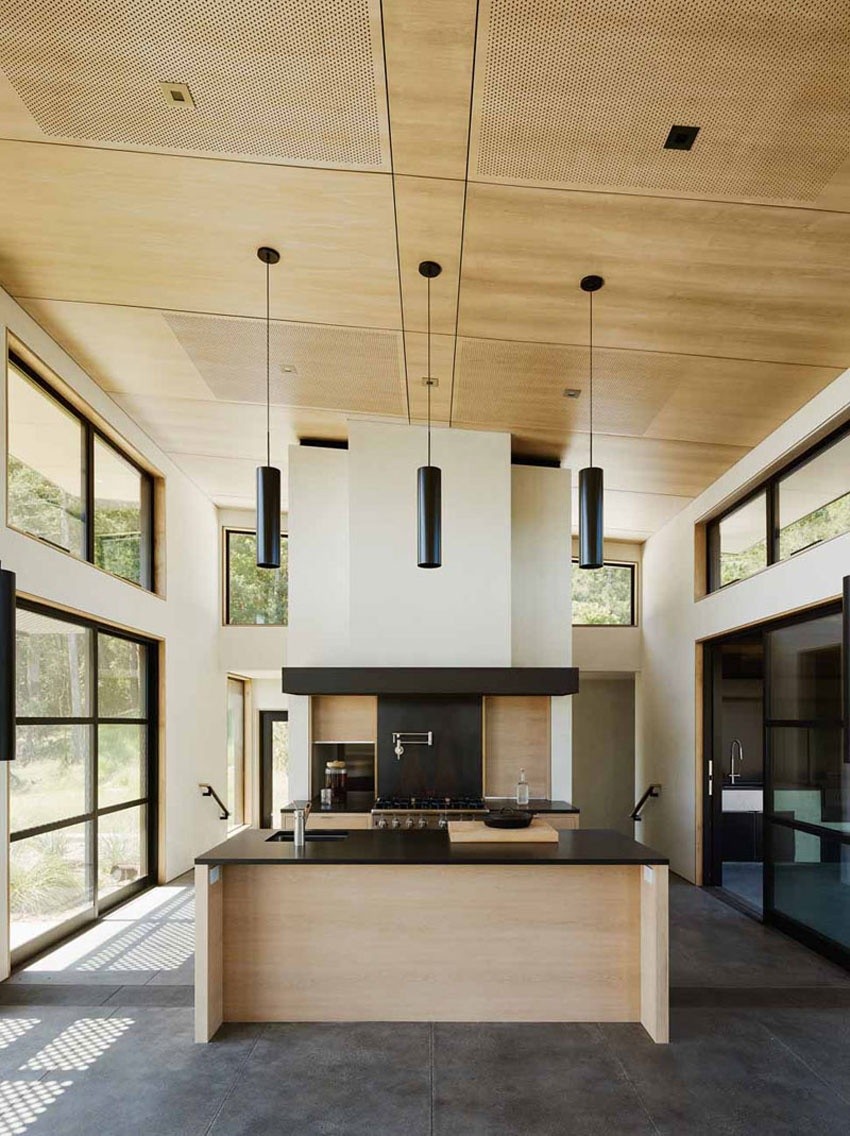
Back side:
[730,739,743,784]
[293,802,312,847]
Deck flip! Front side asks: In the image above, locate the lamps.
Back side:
[111,865,137,882]
[256,246,280,569]
[580,275,605,570]
[418,261,442,568]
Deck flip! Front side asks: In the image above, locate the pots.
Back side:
[484,806,533,828]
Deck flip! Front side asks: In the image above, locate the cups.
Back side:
[320,789,331,805]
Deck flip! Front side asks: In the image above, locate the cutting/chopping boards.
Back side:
[448,818,560,843]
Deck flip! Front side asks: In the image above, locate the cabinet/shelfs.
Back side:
[533,817,576,830]
[725,810,796,863]
[285,816,368,830]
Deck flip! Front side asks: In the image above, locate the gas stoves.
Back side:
[371,794,489,831]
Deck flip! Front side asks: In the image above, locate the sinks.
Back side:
[722,784,762,790]
[265,830,349,842]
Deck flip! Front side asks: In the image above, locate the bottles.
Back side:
[517,768,529,804]
[325,760,347,802]
